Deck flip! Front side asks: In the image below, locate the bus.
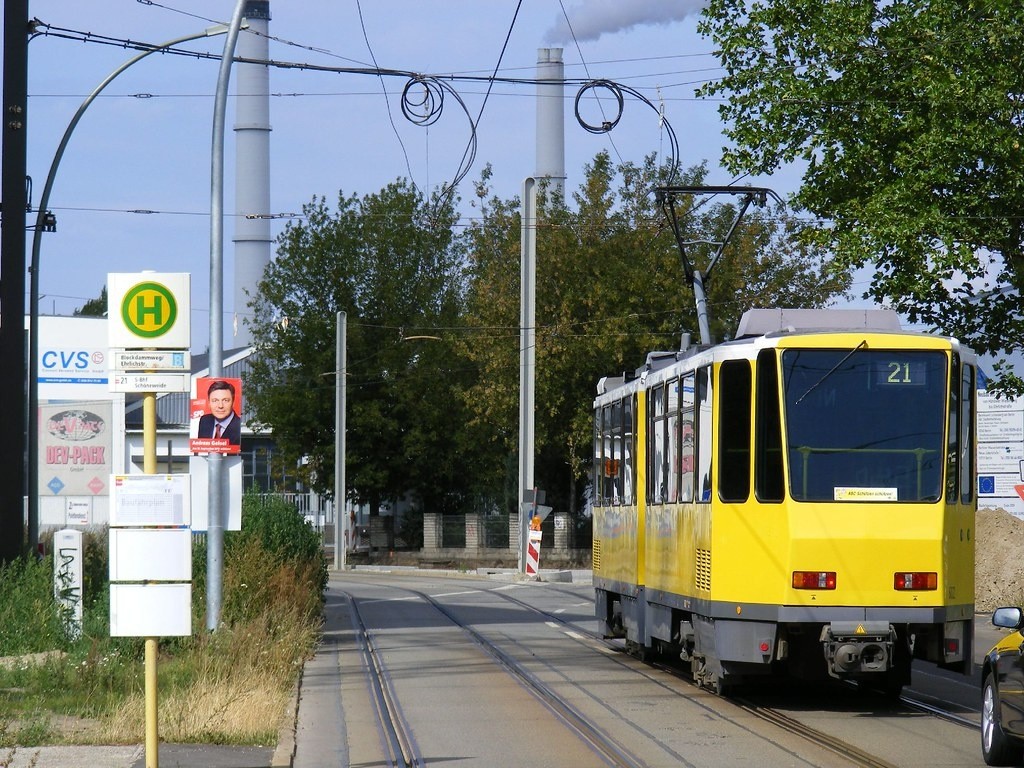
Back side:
[593,304,979,707]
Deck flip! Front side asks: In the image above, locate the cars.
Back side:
[980,607,1024,768]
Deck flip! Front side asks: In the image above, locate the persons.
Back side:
[198,381,241,447]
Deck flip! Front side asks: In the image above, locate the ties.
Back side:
[214,424,221,438]
